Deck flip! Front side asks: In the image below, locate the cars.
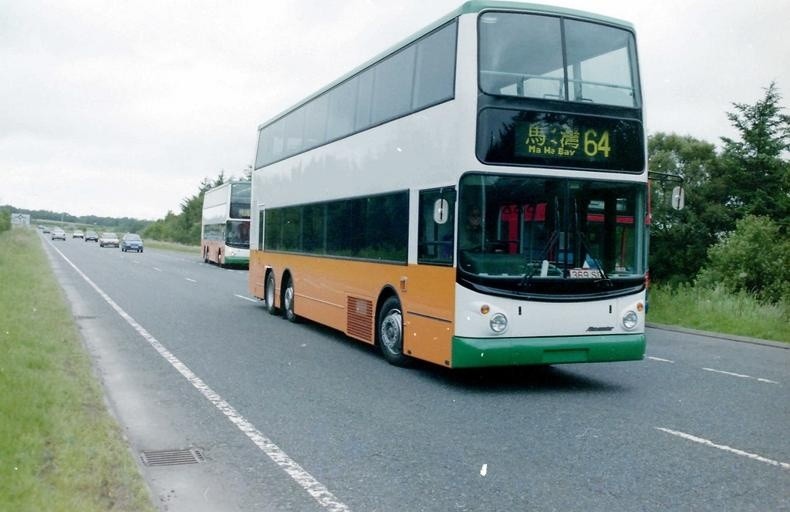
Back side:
[38,225,143,252]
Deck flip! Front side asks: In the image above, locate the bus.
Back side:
[249,0,684,369]
[201,181,251,269]
